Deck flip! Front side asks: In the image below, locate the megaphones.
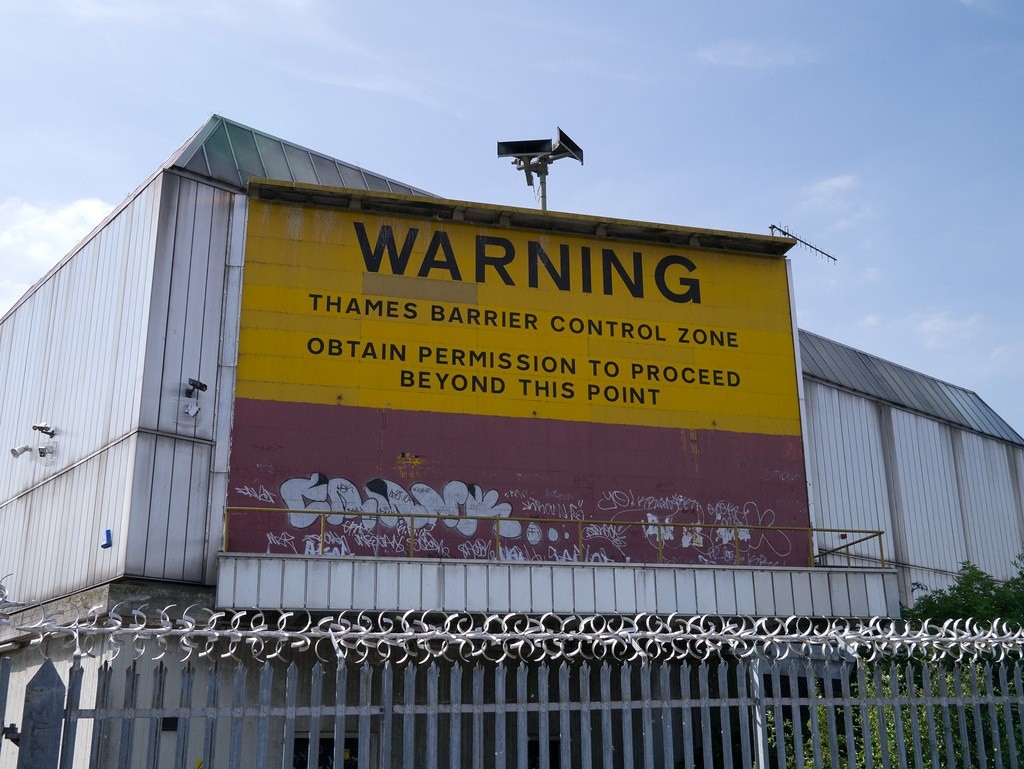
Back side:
[497,127,584,170]
